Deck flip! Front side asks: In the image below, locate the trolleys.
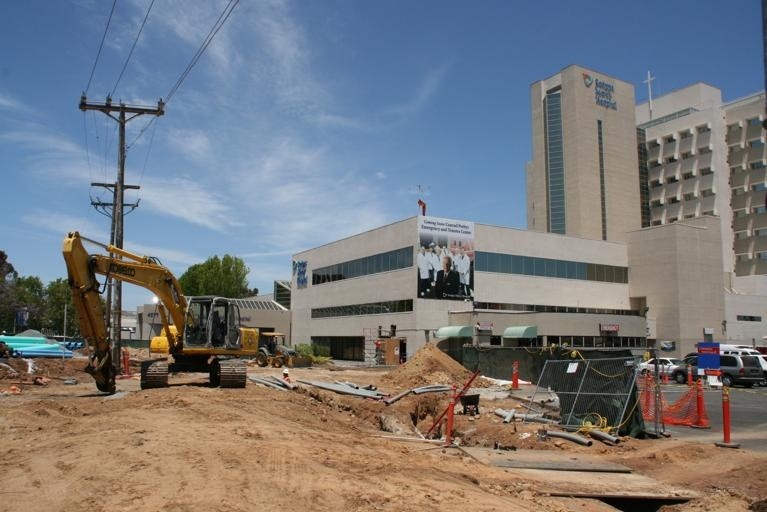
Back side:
[456,394,481,415]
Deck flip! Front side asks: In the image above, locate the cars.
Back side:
[632,343,767,389]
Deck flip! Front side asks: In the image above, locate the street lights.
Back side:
[130,331,136,340]
[13,307,28,334]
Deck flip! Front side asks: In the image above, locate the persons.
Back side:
[269,337,275,349]
[418,243,470,299]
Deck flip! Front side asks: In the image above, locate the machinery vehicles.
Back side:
[62,229,261,398]
[256,331,316,369]
